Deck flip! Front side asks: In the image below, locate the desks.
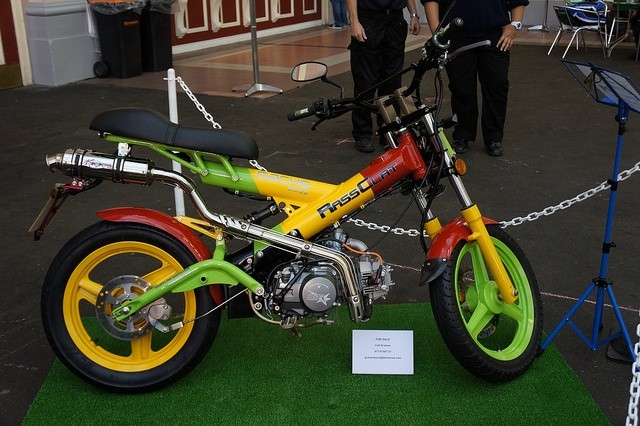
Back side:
[608,0,640,57]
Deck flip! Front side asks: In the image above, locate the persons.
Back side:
[346,1,423,154]
[327,0,352,34]
[419,0,530,156]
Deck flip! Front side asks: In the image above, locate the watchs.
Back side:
[516,22,522,29]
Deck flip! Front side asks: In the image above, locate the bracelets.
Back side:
[409,13,422,19]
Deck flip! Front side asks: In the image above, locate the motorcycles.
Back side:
[29,0,543,395]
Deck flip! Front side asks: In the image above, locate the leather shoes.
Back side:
[486,141,504,156]
[452,139,468,153]
[355,138,375,152]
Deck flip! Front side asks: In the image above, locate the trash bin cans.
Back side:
[88,0,147,78]
[140,0,177,72]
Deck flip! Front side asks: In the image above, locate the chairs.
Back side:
[609,9,639,45]
[566,1,612,46]
[547,6,609,58]
